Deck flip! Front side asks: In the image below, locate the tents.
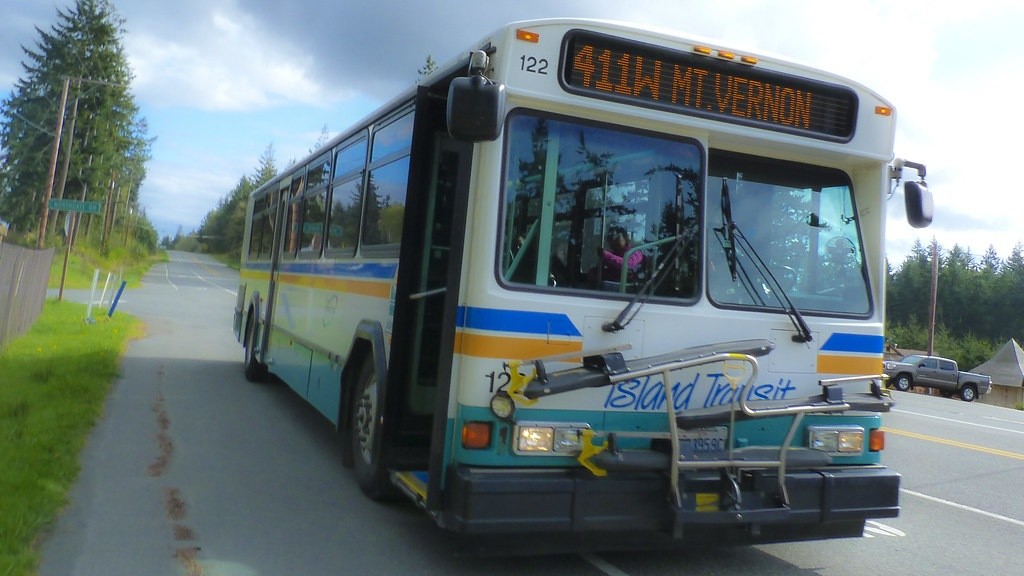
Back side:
[970,338,1024,387]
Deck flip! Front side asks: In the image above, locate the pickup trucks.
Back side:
[883,353,993,403]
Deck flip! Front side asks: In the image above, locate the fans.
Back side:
[826,237,857,266]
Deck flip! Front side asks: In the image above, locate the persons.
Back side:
[601,226,644,283]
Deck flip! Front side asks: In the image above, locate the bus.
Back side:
[232,18,936,561]
